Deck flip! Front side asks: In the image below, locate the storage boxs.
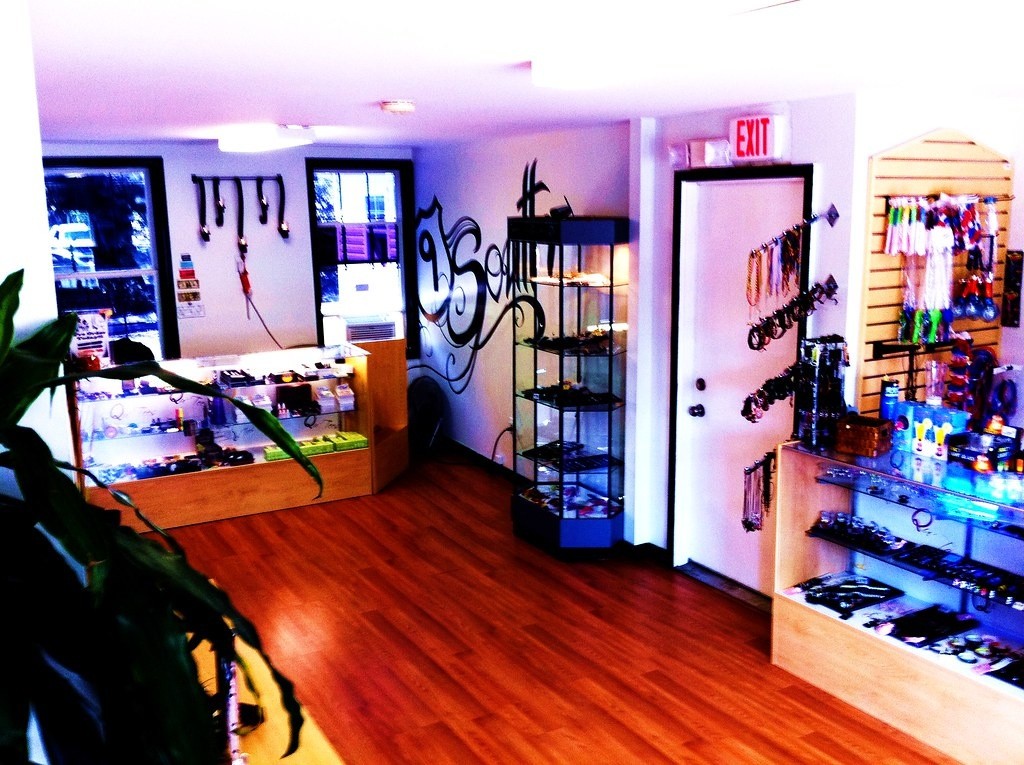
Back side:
[234,394,274,424]
[316,383,356,414]
[220,368,266,389]
[262,431,368,461]
[946,430,1021,476]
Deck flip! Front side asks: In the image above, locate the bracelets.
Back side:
[740,281,837,423]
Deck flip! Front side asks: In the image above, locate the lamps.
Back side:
[218,124,314,153]
[667,136,733,169]
[381,98,415,112]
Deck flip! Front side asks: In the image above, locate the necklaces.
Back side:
[748,230,802,306]
[741,452,776,530]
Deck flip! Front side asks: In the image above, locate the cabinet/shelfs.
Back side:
[770,439,1024,765]
[507,215,632,550]
[64,336,410,535]
